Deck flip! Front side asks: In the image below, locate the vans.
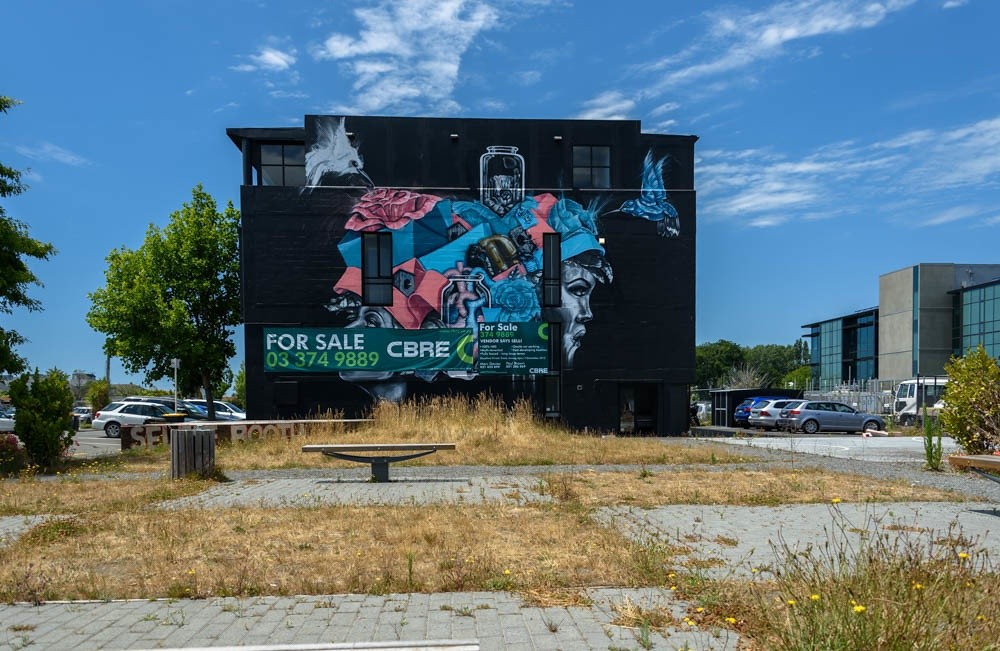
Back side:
[696,402,709,421]
[71,406,93,424]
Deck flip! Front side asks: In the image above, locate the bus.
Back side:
[890,375,958,427]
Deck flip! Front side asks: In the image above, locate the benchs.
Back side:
[302,443,456,484]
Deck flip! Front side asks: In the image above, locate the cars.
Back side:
[0,406,17,433]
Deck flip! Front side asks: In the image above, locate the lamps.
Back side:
[449,133,460,145]
[345,132,356,148]
[598,224,606,244]
[551,135,563,146]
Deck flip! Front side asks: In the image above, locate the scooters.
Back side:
[690,400,701,427]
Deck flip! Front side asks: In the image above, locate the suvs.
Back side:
[91,396,247,438]
[734,395,886,434]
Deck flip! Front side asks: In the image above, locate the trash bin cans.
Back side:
[170,429,216,480]
